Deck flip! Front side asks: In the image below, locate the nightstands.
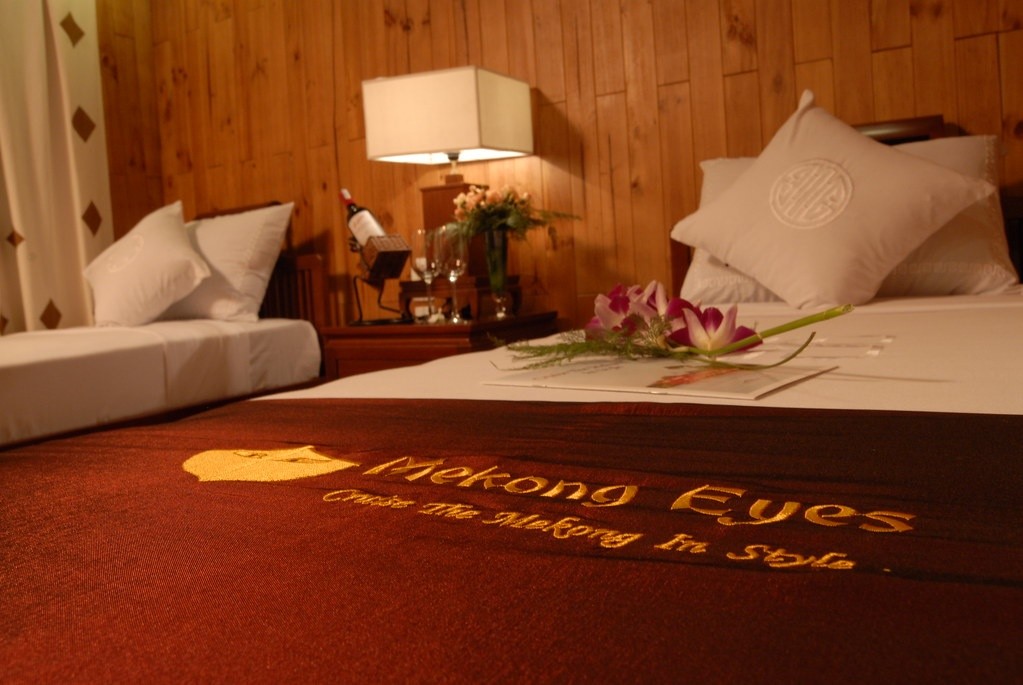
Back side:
[319,309,560,381]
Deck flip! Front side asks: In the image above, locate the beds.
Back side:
[0,254,322,449]
[0,114,1023,684]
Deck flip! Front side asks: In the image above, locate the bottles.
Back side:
[339,188,387,248]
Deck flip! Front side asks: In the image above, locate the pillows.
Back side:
[161,202,296,322]
[679,135,1018,306]
[81,200,211,327]
[669,89,996,318]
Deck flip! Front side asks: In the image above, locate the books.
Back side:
[480,356,840,401]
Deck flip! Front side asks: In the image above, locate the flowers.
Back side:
[485,281,849,378]
[440,182,582,252]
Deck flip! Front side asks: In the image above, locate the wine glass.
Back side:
[433,223,471,323]
[412,230,444,324]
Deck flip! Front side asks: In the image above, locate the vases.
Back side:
[484,230,516,321]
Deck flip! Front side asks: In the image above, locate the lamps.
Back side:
[362,64,534,318]
